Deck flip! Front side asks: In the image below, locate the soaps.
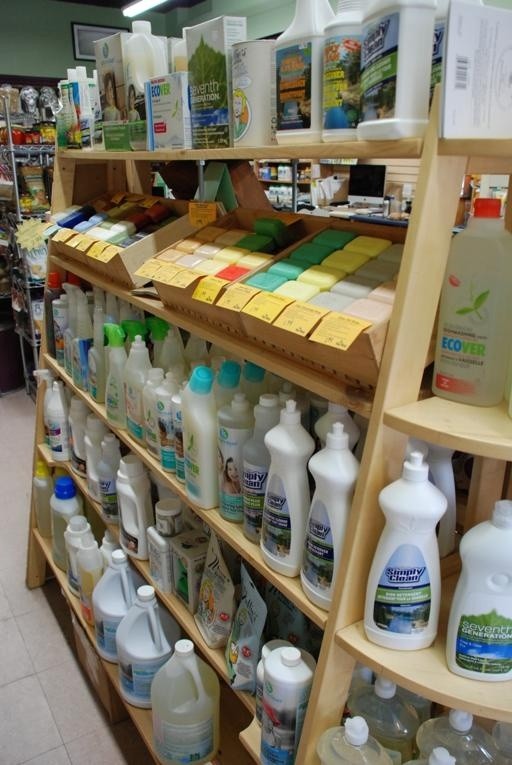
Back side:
[53,190,406,391]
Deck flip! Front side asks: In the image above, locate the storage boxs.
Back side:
[170,527,212,614]
[145,203,337,347]
[49,190,198,291]
[222,220,450,396]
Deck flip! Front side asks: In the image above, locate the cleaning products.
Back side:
[316,663,512,765]
[430,198,512,408]
[40,277,363,616]
[362,423,512,685]
[30,365,320,765]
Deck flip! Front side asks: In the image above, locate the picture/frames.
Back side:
[67,18,131,62]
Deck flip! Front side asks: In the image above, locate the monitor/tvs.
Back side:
[346,164,387,212]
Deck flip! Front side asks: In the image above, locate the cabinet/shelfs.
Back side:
[252,157,355,209]
[20,133,442,765]
[293,135,512,763]
[0,95,53,407]
